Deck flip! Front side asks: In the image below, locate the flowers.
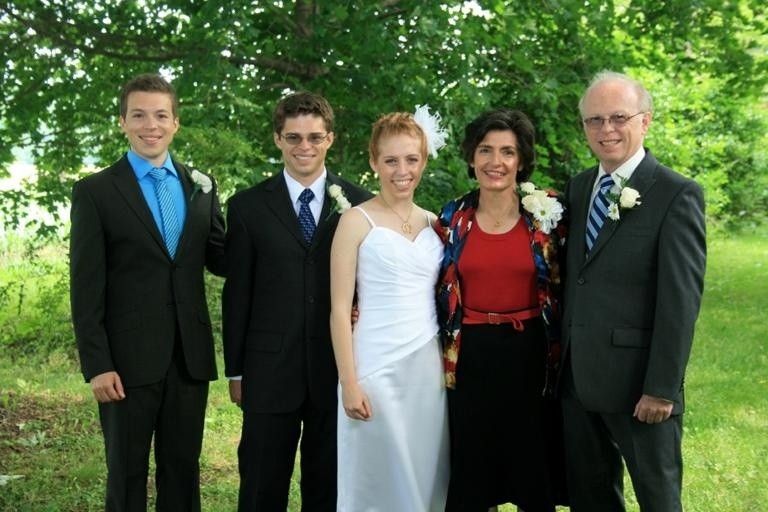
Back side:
[602,178,644,222]
[512,180,564,235]
[322,179,353,223]
[184,168,214,202]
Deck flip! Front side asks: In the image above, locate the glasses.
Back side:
[582,111,644,128]
[277,133,328,145]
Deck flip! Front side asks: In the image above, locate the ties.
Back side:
[296,189,317,244]
[584,174,614,259]
[149,166,184,259]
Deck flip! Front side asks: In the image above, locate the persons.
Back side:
[350,110,570,512]
[329,112,451,512]
[555,73,707,511]
[69,74,226,512]
[221,91,376,512]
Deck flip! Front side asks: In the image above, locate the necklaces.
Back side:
[379,192,414,236]
[480,191,518,228]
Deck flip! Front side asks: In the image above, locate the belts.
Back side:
[458,307,540,331]
